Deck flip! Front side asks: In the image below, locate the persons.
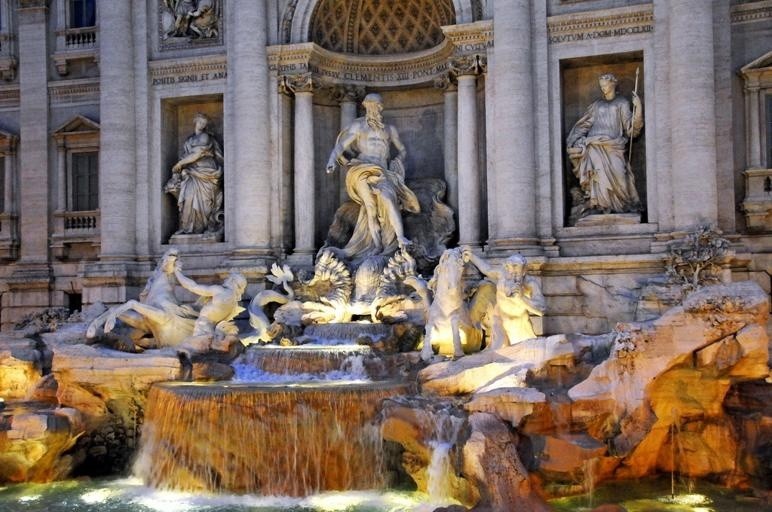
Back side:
[566,73,643,216]
[169,257,247,351]
[460,247,549,354]
[162,114,223,239]
[324,91,416,257]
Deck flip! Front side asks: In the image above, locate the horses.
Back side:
[84,243,200,349]
[402,244,498,362]
